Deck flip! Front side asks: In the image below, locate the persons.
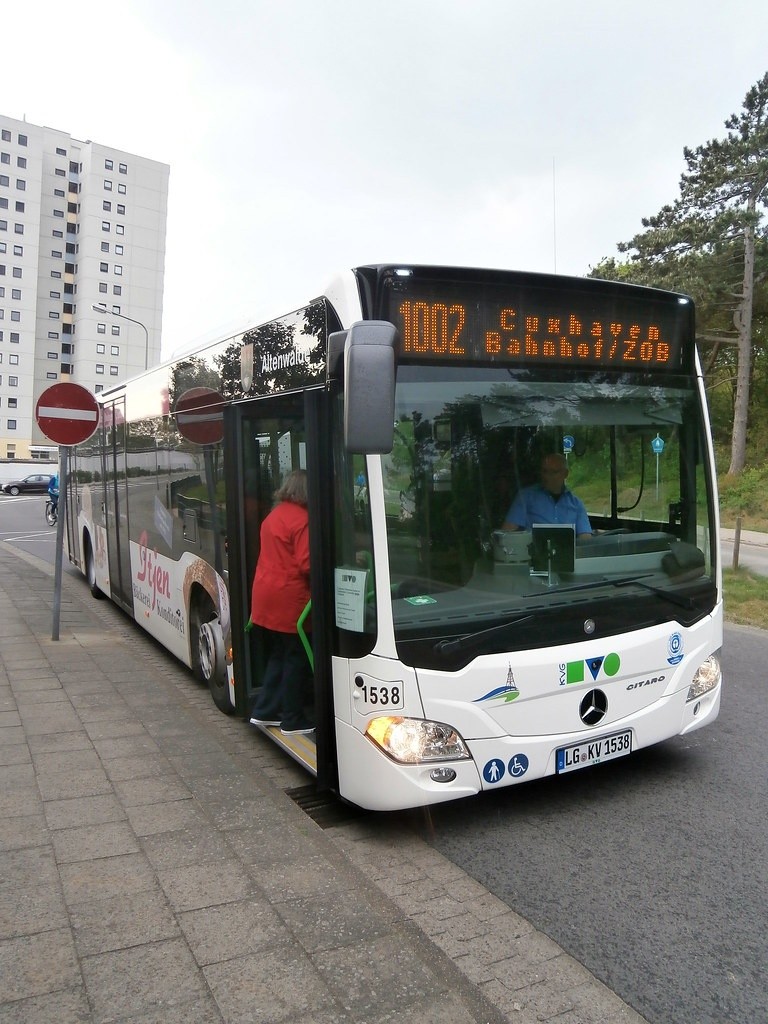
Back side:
[250,468,316,736]
[355,471,367,497]
[501,454,594,539]
[48,471,60,520]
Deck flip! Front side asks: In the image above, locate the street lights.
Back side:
[90,304,149,369]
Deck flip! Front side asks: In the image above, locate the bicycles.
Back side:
[45,498,58,527]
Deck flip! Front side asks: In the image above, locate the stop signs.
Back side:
[36,382,100,447]
[176,386,227,447]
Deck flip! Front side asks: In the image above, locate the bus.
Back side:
[62,261,721,814]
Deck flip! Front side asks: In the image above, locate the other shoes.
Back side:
[50,512,55,518]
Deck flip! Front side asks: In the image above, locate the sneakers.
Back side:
[249,714,315,736]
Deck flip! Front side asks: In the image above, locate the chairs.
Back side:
[471,430,539,564]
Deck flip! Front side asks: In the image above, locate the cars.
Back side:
[0,473,54,495]
